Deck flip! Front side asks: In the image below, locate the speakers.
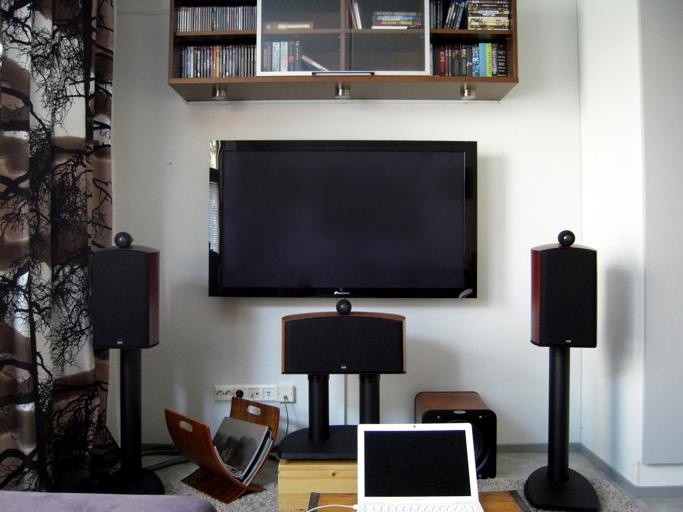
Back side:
[530,243,599,349]
[414,392,498,479]
[281,311,407,375]
[89,245,160,350]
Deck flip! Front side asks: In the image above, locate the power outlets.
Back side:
[261,386,276,401]
[235,386,248,401]
[277,386,293,403]
[248,386,261,402]
[214,384,225,401]
[225,386,233,400]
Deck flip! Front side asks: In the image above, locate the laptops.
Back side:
[358,422,486,511]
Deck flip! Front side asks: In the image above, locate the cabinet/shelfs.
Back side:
[278,464,358,512]
[343,1,519,100]
[169,0,340,102]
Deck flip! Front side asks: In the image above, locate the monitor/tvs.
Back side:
[209,139,478,300]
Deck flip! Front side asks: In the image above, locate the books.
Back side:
[349,0,362,30]
[263,22,314,32]
[466,0,509,30]
[372,11,422,30]
[431,43,506,77]
[301,54,329,72]
[182,45,256,79]
[444,1,464,29]
[177,7,256,31]
[429,0,443,30]
[261,41,302,72]
[212,417,274,486]
